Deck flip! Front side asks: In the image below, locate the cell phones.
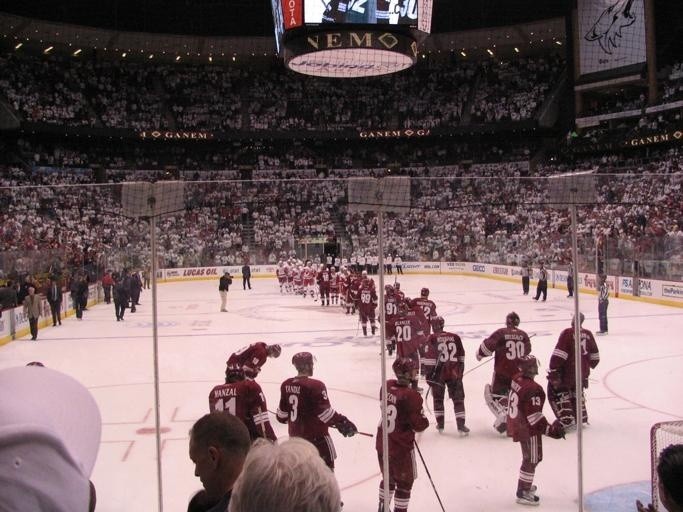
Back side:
[229,276,234,278]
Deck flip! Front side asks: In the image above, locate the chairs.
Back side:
[516,489,539,502]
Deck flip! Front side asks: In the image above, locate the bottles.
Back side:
[0,365,101,512]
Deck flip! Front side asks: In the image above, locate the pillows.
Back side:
[505,312,520,327]
[385,282,444,326]
[519,355,540,377]
[393,358,417,378]
[225,345,313,374]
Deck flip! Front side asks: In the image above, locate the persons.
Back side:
[219,273,232,312]
[242,263,251,290]
[566,62,683,139]
[597,273,609,335]
[547,313,600,429]
[2,53,567,131]
[506,355,566,507]
[106,176,403,274]
[276,258,317,298]
[23,287,43,340]
[565,260,575,297]
[532,261,548,301]
[407,288,438,375]
[46,279,63,326]
[386,147,682,283]
[387,304,428,393]
[228,436,343,511]
[103,267,143,321]
[1,169,107,310]
[142,266,151,289]
[276,352,358,473]
[71,274,90,319]
[209,369,277,443]
[376,357,429,511]
[316,265,334,307]
[354,279,377,336]
[329,267,368,315]
[421,316,470,437]
[2,139,545,188]
[597,263,603,291]
[476,313,532,436]
[636,445,683,511]
[225,342,282,378]
[2,365,102,512]
[187,411,252,511]
[378,282,404,355]
[520,261,530,295]
